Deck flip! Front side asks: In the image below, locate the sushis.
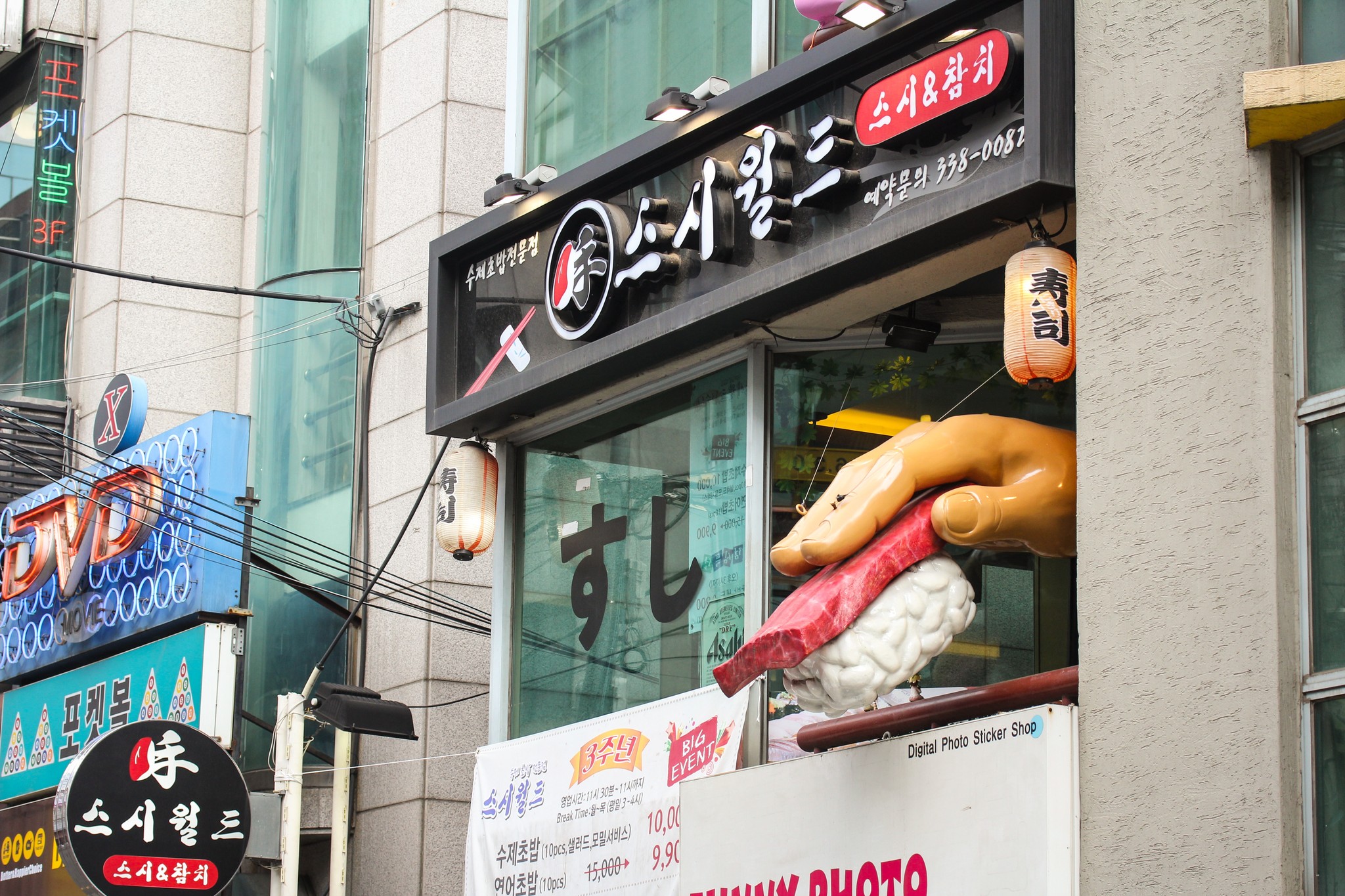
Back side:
[711,485,977,717]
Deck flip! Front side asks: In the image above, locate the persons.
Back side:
[770,414,1078,577]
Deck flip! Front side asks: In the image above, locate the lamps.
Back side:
[833,0,905,30]
[484,173,541,209]
[882,302,941,354]
[644,86,711,123]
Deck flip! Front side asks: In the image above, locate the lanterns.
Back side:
[1003,246,1076,388]
[434,440,500,564]
